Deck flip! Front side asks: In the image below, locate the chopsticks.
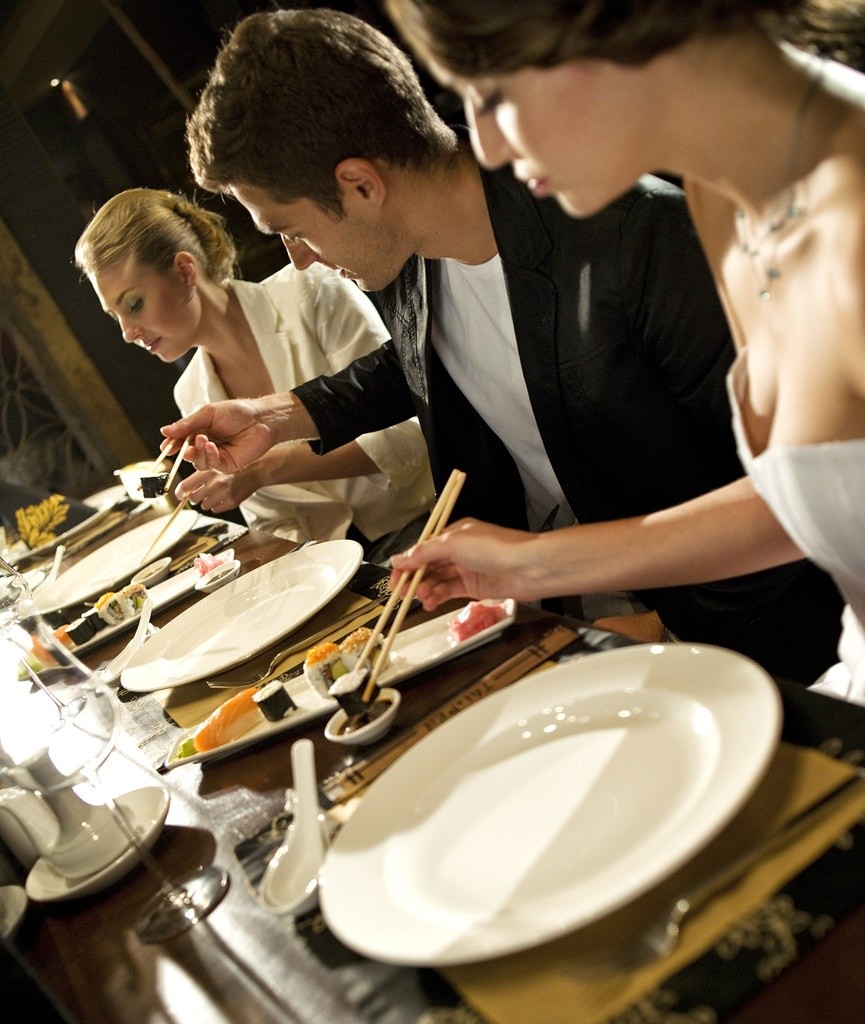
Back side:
[136,432,197,492]
[350,470,466,702]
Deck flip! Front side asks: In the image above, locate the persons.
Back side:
[73,186,438,567]
[159,8,844,690]
[388,0,865,711]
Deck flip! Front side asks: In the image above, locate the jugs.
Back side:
[0,557,152,791]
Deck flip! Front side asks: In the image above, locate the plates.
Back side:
[325,687,401,743]
[318,643,779,965]
[23,570,46,590]
[19,549,235,685]
[6,485,127,568]
[164,596,513,777]
[120,539,364,692]
[0,887,27,942]
[27,785,169,900]
[22,509,199,615]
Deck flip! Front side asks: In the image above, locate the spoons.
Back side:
[256,740,329,918]
[33,545,66,597]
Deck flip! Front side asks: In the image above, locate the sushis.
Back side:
[303,642,355,700]
[327,668,380,716]
[140,472,171,499]
[29,582,149,667]
[338,626,392,676]
[191,686,266,753]
[251,679,297,721]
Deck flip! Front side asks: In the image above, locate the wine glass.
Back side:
[9,642,86,732]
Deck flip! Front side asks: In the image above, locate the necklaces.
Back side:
[731,70,821,301]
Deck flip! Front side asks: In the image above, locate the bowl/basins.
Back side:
[119,460,173,502]
